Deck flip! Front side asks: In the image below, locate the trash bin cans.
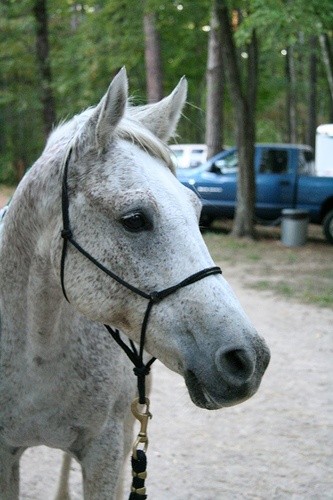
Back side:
[280,206,310,250]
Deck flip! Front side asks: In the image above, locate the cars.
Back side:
[178,141,333,244]
[168,146,219,166]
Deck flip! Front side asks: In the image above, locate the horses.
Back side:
[1,64,270,500]
